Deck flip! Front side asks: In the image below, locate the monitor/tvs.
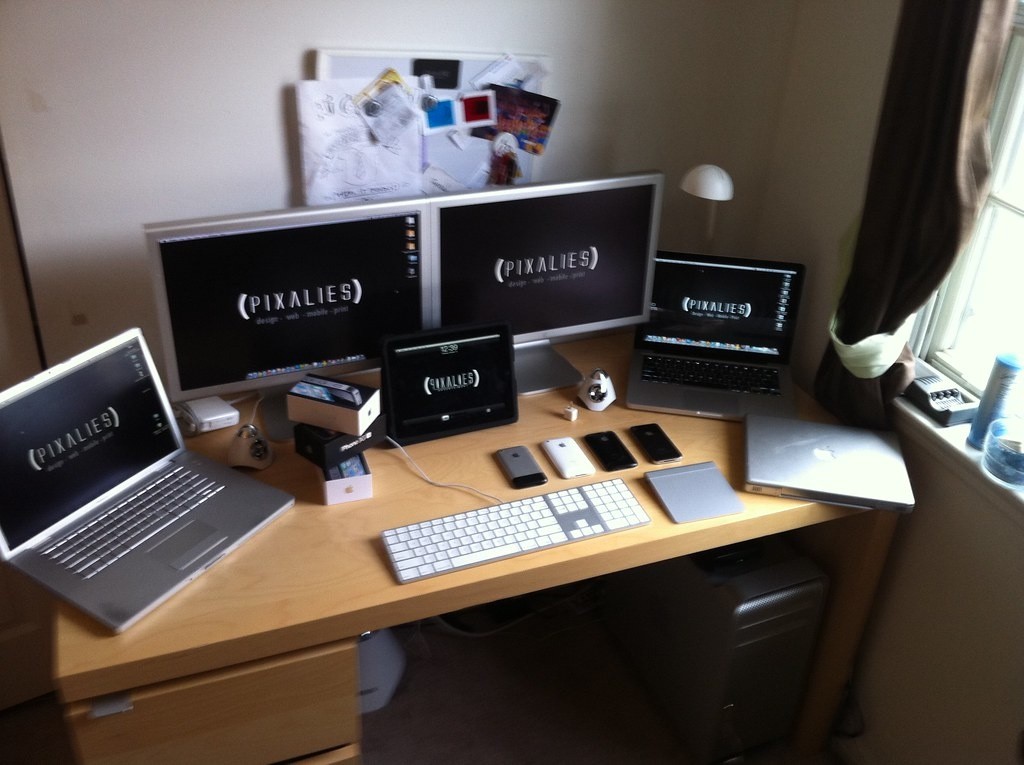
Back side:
[144,170,667,403]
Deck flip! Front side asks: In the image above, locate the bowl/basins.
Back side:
[982,417,1024,492]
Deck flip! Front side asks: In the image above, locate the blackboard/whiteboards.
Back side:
[313,47,551,197]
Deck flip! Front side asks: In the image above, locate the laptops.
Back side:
[623,252,807,424]
[0,328,295,633]
[742,412,915,514]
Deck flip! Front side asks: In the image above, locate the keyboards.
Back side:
[379,479,650,585]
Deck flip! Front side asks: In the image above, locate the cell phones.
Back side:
[630,423,684,466]
[496,446,548,488]
[541,436,596,480]
[584,431,637,471]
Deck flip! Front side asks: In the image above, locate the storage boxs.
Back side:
[294,412,387,469]
[315,452,375,508]
[286,372,380,436]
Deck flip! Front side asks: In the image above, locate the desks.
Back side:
[49,329,915,764]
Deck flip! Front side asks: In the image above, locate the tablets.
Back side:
[378,319,520,448]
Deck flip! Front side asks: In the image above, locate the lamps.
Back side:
[679,163,734,254]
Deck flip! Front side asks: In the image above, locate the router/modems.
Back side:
[180,395,240,432]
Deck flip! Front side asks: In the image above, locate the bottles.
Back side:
[965,350,1024,452]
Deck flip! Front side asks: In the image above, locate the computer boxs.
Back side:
[591,533,831,761]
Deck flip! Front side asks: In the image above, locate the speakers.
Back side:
[227,423,273,472]
[575,366,617,412]
